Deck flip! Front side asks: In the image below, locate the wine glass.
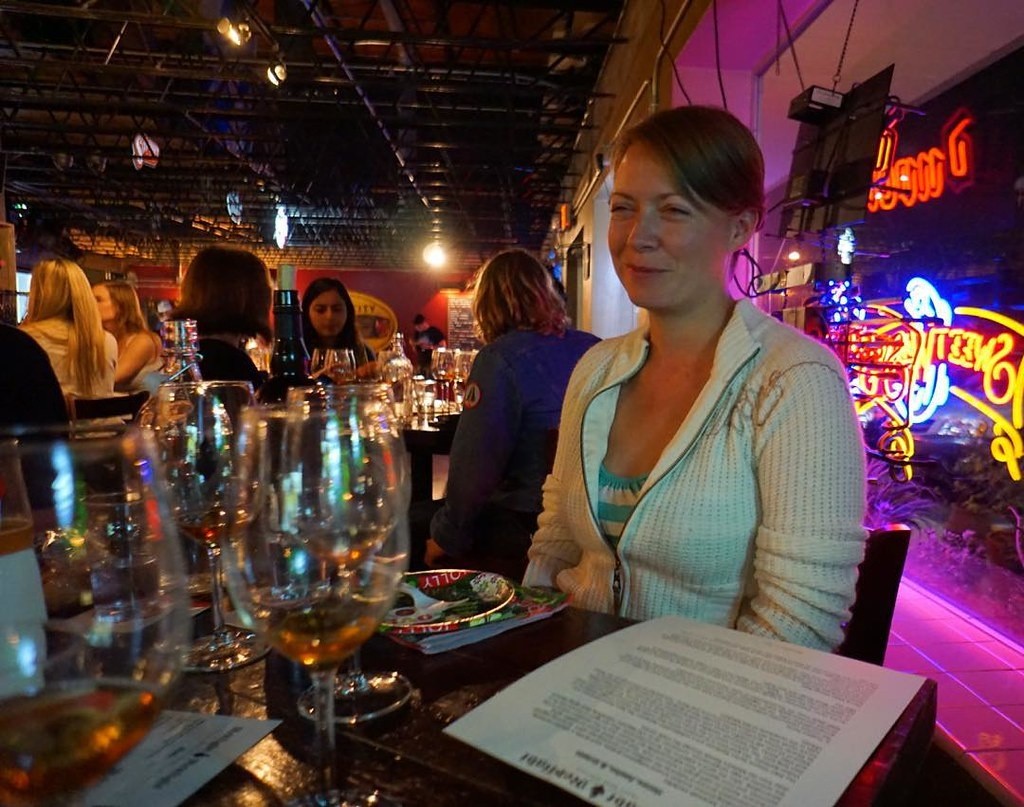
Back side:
[0,348,481,806]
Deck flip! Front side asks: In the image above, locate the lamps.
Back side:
[220,6,289,86]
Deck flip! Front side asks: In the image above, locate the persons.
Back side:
[519,102,870,657]
[0,325,69,437]
[166,244,274,397]
[408,314,447,377]
[92,280,163,394]
[16,256,118,400]
[272,277,377,381]
[424,248,605,577]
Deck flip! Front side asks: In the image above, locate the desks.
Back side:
[262,396,463,572]
[48,592,938,806]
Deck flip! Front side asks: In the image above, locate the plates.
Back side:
[378,568,516,635]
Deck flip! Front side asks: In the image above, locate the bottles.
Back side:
[384,333,412,380]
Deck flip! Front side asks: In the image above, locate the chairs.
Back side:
[67,389,150,429]
